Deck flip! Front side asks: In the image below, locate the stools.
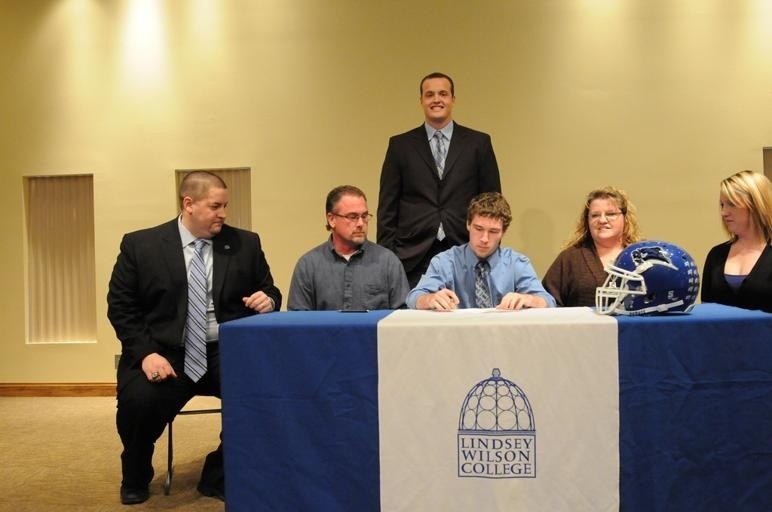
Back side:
[163,386,233,492]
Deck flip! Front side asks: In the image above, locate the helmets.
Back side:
[594,240,699,316]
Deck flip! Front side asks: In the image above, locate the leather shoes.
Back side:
[121,464,154,504]
[197,469,225,501]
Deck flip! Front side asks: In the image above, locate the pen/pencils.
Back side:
[341,310,368,313]
[439,286,453,299]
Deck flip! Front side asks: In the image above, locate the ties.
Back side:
[475,261,491,308]
[184,240,208,383]
[434,131,446,243]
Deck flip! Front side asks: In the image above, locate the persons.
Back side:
[108,171,282,505]
[288,186,409,311]
[701,170,771,313]
[379,73,502,309]
[407,194,555,312]
[539,188,648,308]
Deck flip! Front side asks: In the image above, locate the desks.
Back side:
[220,305,772,511]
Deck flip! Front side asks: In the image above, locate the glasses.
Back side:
[589,211,623,220]
[334,213,373,223]
